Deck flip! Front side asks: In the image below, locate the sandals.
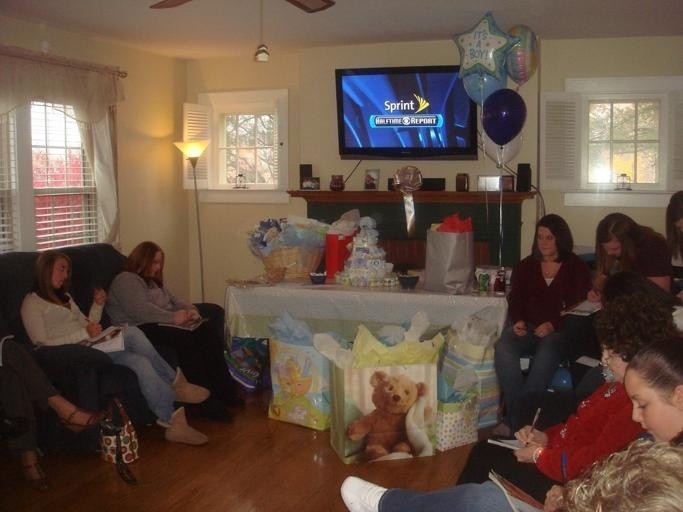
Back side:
[17,461,48,490]
[59,404,108,438]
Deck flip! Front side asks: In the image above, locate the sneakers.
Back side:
[340,475,390,512]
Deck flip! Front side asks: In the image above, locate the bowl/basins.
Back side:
[265,265,286,282]
[397,274,420,288]
[308,272,327,283]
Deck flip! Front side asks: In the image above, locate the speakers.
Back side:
[516,163,532,191]
[299,164,312,178]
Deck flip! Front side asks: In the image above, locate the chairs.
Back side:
[556,251,597,368]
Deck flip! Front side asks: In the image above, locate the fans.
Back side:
[149,0,336,14]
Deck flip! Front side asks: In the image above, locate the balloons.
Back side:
[454,11,521,82]
[504,25,540,84]
[481,91,527,146]
[462,74,506,105]
[481,131,524,167]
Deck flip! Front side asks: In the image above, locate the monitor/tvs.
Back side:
[334,64,481,160]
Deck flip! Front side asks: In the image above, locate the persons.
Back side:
[20,250,211,446]
[0,339,109,491]
[106,241,245,419]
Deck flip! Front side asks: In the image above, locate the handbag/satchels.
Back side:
[96,396,140,465]
[326,351,440,465]
[266,337,334,431]
[422,229,479,297]
[439,331,506,432]
[325,229,359,280]
[436,393,482,453]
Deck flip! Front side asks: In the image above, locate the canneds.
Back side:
[301,176,320,191]
[364,169,379,192]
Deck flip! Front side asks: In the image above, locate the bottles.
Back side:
[494,265,507,297]
[303,176,320,191]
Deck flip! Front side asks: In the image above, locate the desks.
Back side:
[222,276,510,391]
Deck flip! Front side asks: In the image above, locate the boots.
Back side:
[154,405,208,446]
[168,364,210,404]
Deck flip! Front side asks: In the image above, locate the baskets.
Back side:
[260,245,324,279]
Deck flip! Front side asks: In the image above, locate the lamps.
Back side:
[254,0,270,62]
[172,136,212,304]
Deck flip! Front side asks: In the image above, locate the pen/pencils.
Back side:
[561,451,567,480]
[517,327,536,333]
[591,286,599,296]
[525,407,541,448]
[85,319,94,325]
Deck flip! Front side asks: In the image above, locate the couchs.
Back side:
[0,241,225,460]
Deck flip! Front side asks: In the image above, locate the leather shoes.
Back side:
[196,389,246,422]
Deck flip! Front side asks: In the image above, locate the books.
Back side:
[0,334,14,366]
[159,317,209,332]
[84,325,126,351]
